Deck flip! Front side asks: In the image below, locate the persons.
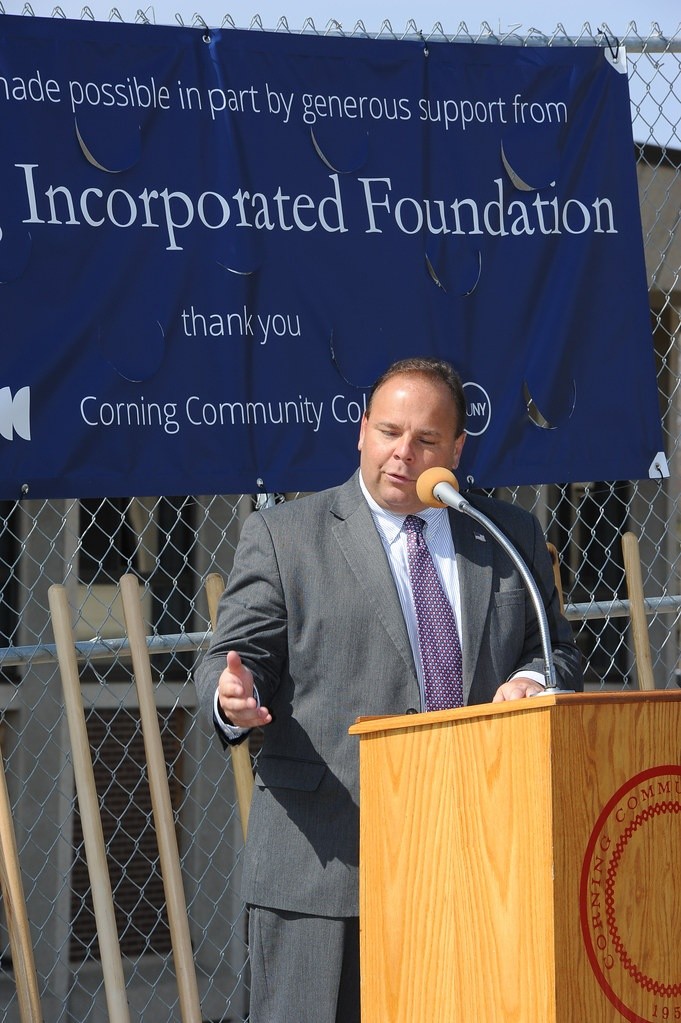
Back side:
[194,361,582,1022]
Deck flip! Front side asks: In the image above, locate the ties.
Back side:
[403,515,465,712]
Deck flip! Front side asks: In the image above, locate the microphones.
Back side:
[415,467,576,697]
